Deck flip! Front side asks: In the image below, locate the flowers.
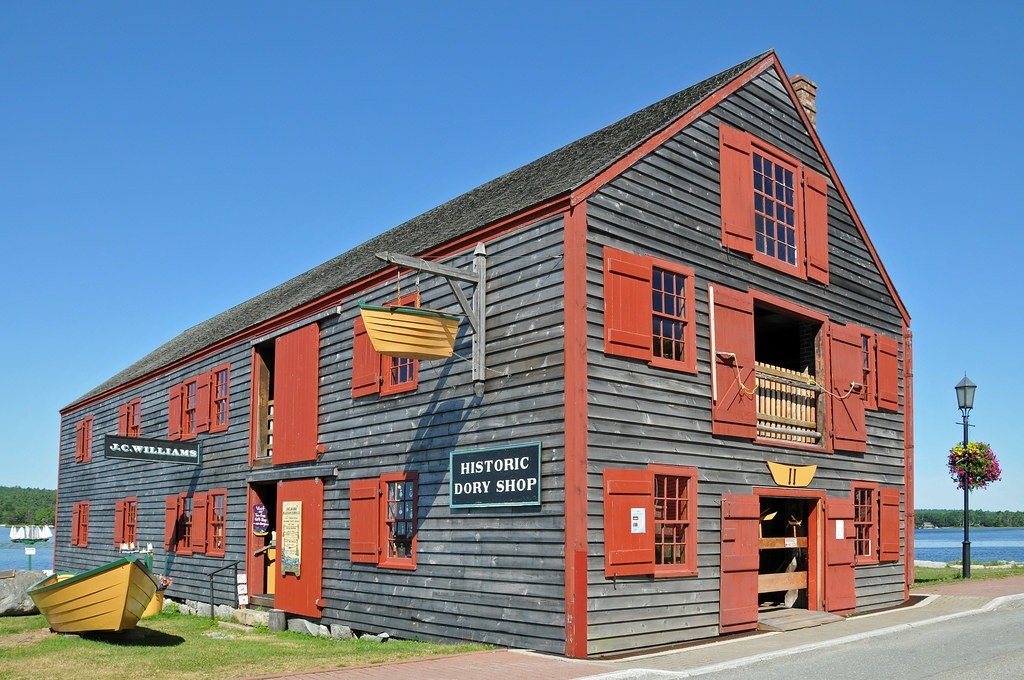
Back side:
[944,441,1004,492]
[152,574,174,591]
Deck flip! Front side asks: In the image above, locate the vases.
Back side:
[141,590,164,618]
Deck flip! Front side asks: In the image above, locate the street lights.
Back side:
[954,372,978,581]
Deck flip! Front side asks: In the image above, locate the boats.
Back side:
[26,556,161,635]
